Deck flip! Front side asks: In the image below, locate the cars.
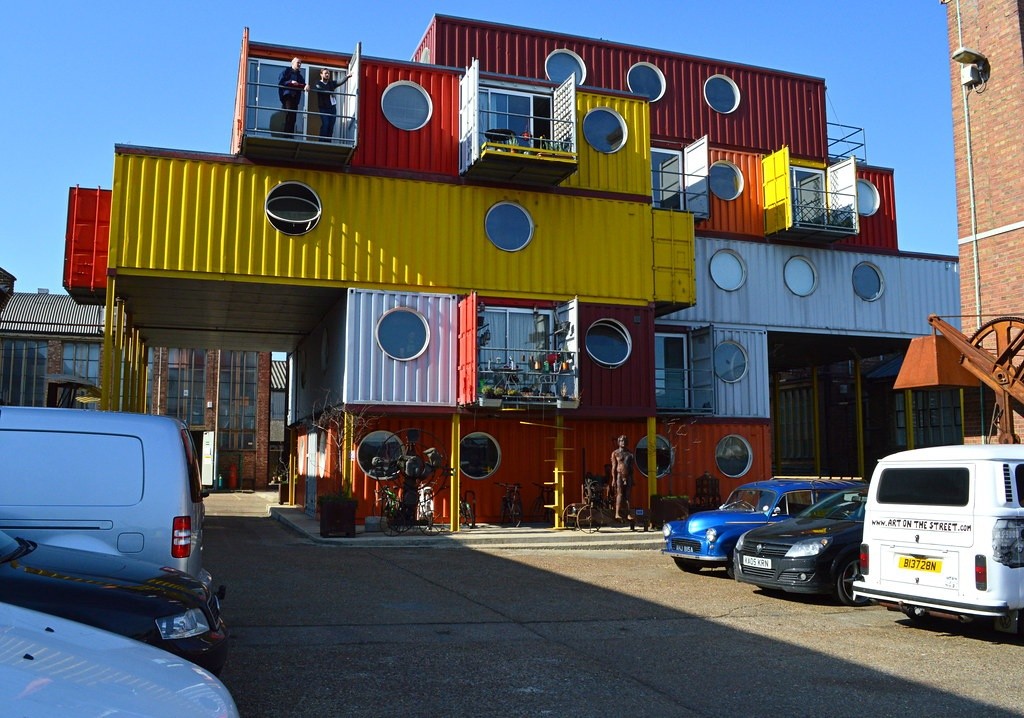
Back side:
[0,530,229,675]
[0,602,241,718]
[733,490,871,606]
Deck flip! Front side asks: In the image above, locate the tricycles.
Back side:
[560,469,636,533]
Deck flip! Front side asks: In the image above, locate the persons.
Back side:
[278,57,311,140]
[610,435,634,520]
[313,68,353,143]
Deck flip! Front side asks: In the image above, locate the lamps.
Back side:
[952,47,988,91]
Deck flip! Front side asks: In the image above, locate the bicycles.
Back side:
[379,484,559,539]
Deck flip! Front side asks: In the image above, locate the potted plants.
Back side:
[305,385,388,538]
[649,414,702,534]
[486,125,574,158]
[275,451,289,505]
[477,382,569,407]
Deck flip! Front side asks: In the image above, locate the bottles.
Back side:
[535,357,540,369]
[553,360,558,371]
[544,359,549,371]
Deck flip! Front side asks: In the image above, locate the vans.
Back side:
[0,408,205,576]
[852,442,1024,642]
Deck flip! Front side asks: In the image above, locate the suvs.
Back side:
[661,475,871,579]
